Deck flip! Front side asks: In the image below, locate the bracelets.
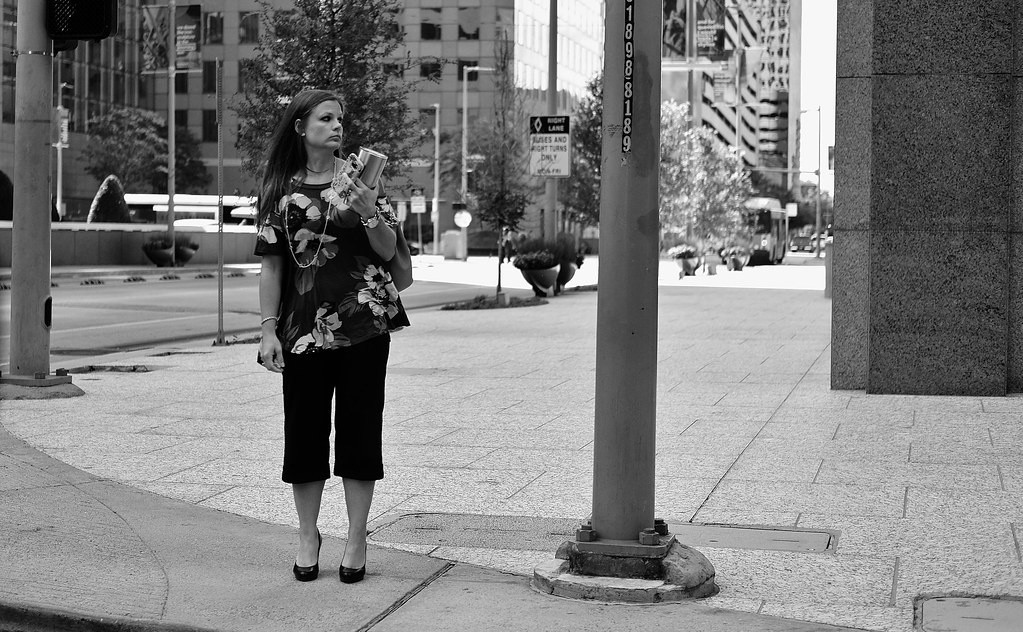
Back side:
[261,317,278,325]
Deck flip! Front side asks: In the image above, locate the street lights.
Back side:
[429,103,440,255]
[461,64,496,261]
[801,106,820,258]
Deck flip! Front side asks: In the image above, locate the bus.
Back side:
[716,197,789,266]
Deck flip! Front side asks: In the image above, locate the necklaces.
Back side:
[285,157,336,268]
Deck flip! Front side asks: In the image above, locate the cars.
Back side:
[790,234,811,252]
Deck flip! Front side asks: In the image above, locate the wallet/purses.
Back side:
[343,147,388,214]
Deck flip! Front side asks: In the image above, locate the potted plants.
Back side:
[720,246,753,271]
[514,234,584,300]
[668,244,703,276]
[142,235,199,267]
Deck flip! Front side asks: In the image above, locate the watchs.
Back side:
[361,207,380,228]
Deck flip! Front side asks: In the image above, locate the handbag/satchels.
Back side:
[388,221,413,292]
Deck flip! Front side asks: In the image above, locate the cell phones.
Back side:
[331,153,364,198]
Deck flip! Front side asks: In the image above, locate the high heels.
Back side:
[339,540,367,584]
[293,527,322,582]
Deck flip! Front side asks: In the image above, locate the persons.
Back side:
[497,226,512,263]
[234,188,240,196]
[254,89,410,583]
